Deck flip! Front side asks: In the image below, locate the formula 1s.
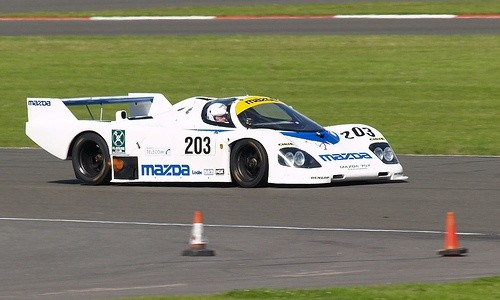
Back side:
[25,89,408,188]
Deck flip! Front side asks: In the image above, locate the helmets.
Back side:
[206,103,228,121]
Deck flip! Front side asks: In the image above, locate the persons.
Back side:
[205,102,232,125]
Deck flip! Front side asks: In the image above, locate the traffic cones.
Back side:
[436,214,468,256]
[181,209,216,258]
